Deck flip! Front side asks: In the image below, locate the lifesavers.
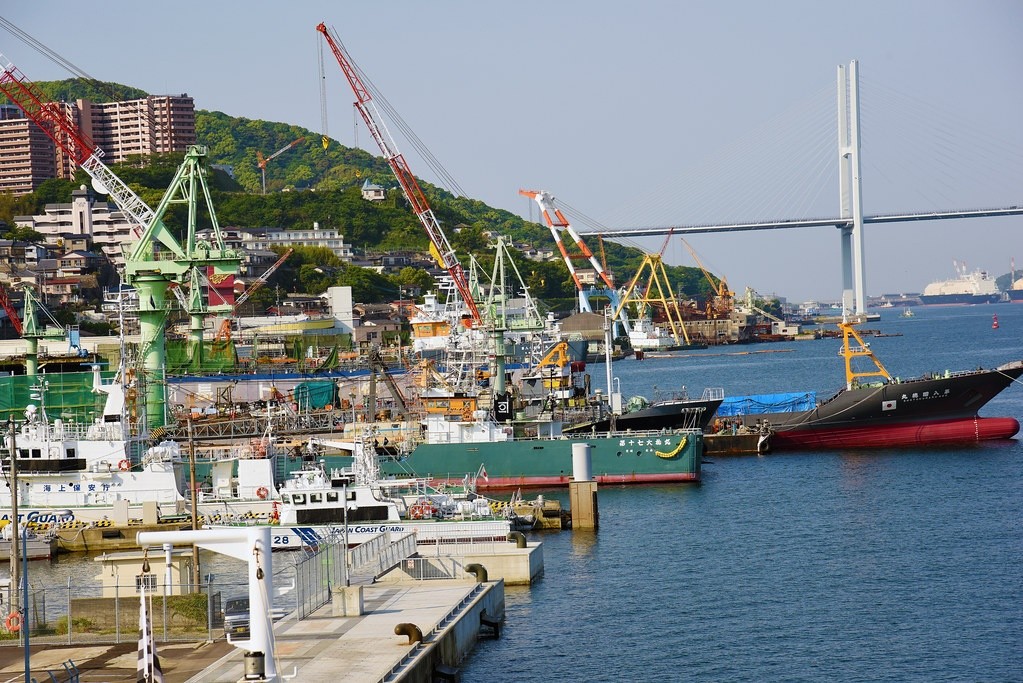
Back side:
[462,411,471,421]
[118,459,130,471]
[256,487,268,498]
[411,506,423,518]
[200,481,213,493]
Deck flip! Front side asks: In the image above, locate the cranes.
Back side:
[0,13,247,334]
[255,137,305,195]
[316,20,515,348]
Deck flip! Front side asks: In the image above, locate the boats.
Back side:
[0,140,1023,551]
